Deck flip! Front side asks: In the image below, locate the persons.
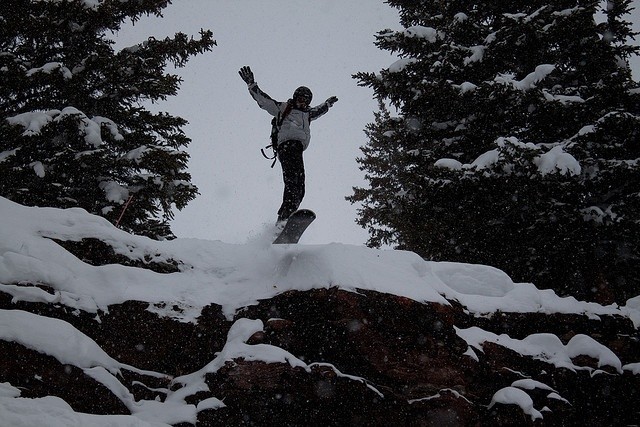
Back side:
[237,64,339,232]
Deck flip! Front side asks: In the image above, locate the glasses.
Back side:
[295,94,312,105]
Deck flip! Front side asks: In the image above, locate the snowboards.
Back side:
[272,209,316,244]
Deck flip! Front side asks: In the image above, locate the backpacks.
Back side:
[260,98,311,167]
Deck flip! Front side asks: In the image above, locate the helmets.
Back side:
[294,85,313,105]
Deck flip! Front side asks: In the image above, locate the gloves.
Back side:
[238,65,254,84]
[326,95,338,107]
